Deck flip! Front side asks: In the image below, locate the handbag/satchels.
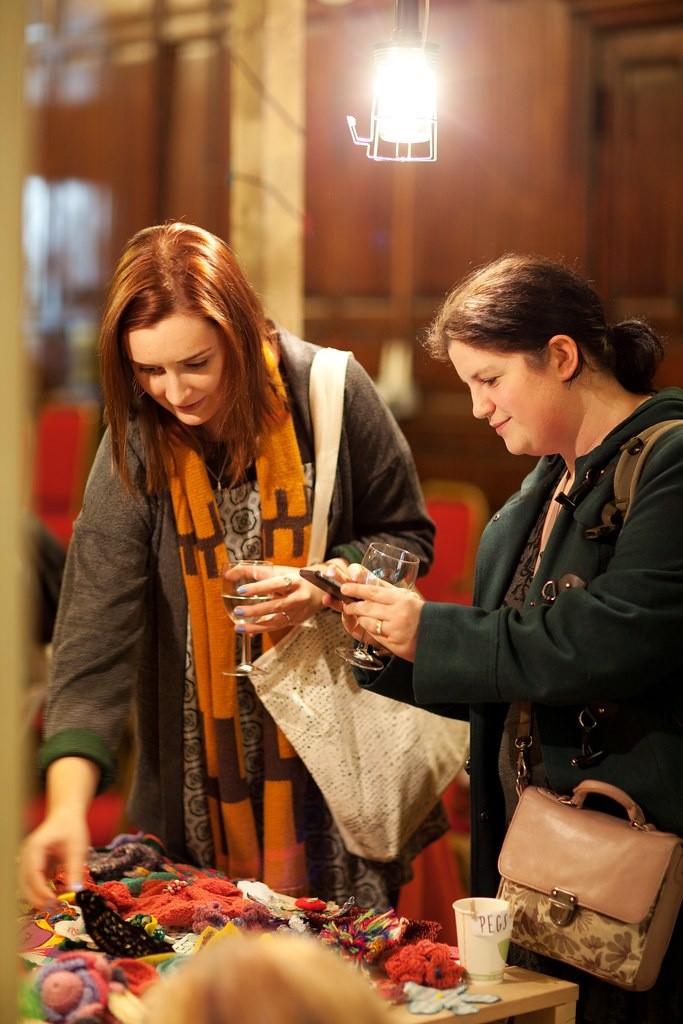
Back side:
[495,785,683,992]
[244,604,472,860]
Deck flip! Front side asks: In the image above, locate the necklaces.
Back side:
[207,453,229,491]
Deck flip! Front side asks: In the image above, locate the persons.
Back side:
[18,215,450,919]
[322,250,683,1024]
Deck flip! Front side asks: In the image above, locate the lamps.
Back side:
[345,0,438,161]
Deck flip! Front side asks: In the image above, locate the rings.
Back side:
[282,611,292,625]
[282,577,292,591]
[377,619,383,636]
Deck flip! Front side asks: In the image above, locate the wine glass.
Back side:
[336,542,420,671]
[220,560,272,676]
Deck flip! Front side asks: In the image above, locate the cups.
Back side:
[452,897,515,985]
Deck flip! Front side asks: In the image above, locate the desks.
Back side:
[18,867,579,1024]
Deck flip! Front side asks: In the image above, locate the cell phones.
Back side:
[299,570,362,604]
